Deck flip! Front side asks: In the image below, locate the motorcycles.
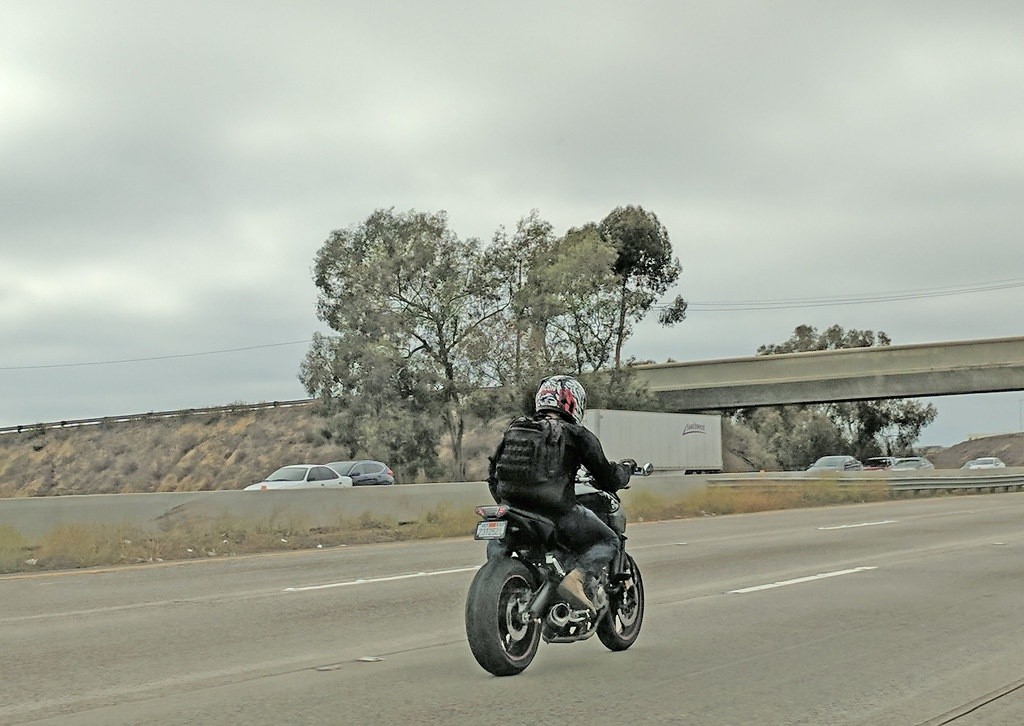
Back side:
[462,462,655,677]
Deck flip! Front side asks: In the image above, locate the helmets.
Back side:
[535,375,587,425]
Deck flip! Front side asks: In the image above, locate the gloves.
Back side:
[621,458,637,475]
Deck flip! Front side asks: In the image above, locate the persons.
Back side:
[489,376,638,617]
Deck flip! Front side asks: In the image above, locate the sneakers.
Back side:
[557,568,597,619]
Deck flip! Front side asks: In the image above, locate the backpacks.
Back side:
[494,415,564,482]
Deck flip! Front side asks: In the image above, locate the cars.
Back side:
[968,456,1005,469]
[322,460,396,487]
[860,457,895,472]
[242,464,352,492]
[805,456,863,473]
[888,456,935,472]
